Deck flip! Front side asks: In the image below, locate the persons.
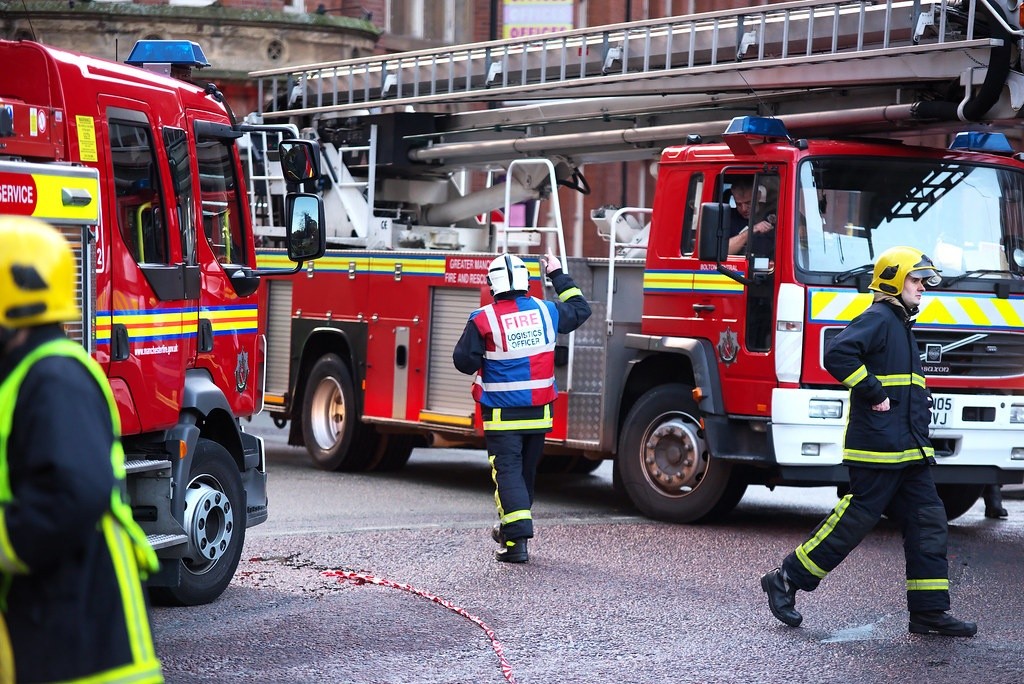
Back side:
[761,245,979,637]
[0,214,166,684]
[727,182,781,349]
[452,246,591,565]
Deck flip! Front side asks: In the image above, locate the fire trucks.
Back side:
[0,32,268,607]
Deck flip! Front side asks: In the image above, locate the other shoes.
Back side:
[907,609,978,637]
[496,538,528,563]
[761,566,803,628]
[491,523,501,542]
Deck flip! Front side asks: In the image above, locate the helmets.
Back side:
[0,214,83,330]
[867,245,943,296]
[486,253,531,296]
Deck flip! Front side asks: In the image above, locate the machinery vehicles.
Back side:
[259,0,1024,526]
